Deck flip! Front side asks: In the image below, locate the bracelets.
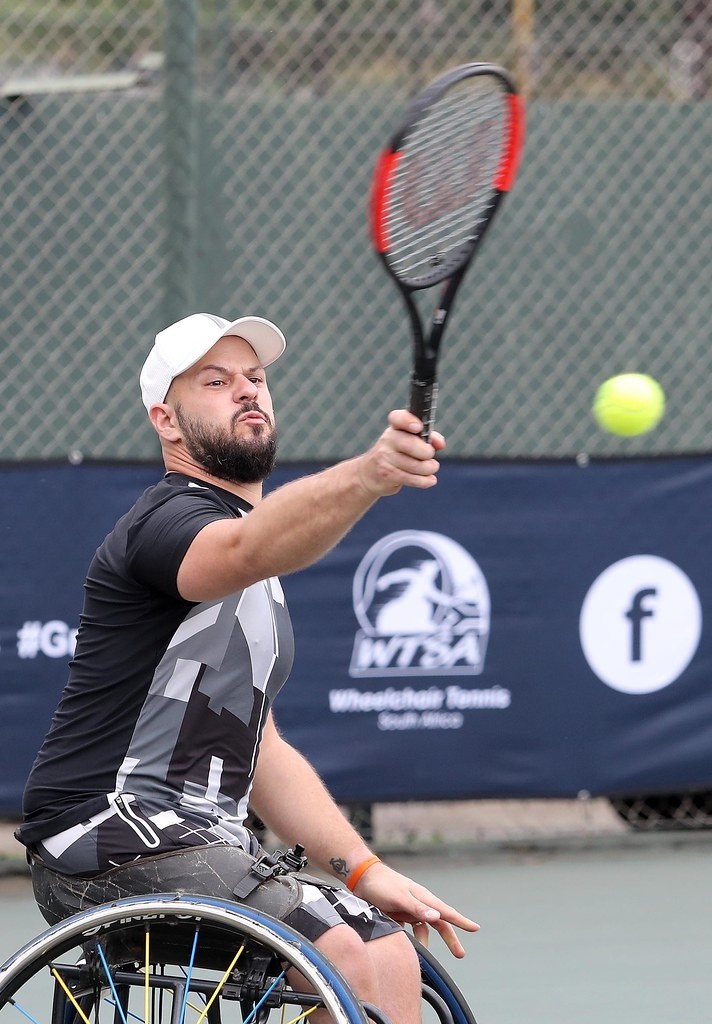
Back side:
[346,855,381,892]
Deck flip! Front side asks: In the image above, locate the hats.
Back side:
[140,313,287,418]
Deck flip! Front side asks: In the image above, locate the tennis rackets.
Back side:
[370,60,524,442]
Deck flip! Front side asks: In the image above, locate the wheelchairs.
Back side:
[0,892,482,1024]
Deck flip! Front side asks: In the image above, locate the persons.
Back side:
[13,312,480,1024]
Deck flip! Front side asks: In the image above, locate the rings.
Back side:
[412,922,426,928]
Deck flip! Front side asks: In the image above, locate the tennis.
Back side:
[595,372,666,436]
